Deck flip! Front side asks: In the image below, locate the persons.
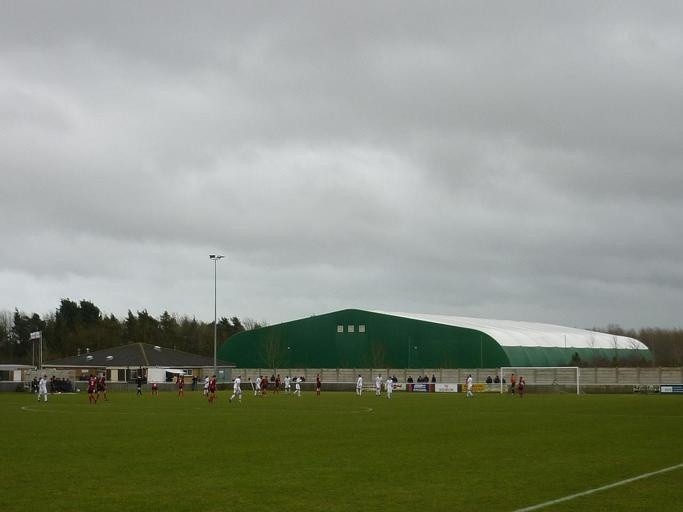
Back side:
[230,373,243,404]
[26,373,73,402]
[136,376,143,396]
[316,373,322,397]
[174,373,218,402]
[465,373,473,398]
[356,373,363,396]
[248,373,305,397]
[79,373,110,407]
[151,382,159,397]
[486,373,525,398]
[407,374,436,383]
[375,373,398,400]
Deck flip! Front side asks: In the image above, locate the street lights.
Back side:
[209,254,226,368]
